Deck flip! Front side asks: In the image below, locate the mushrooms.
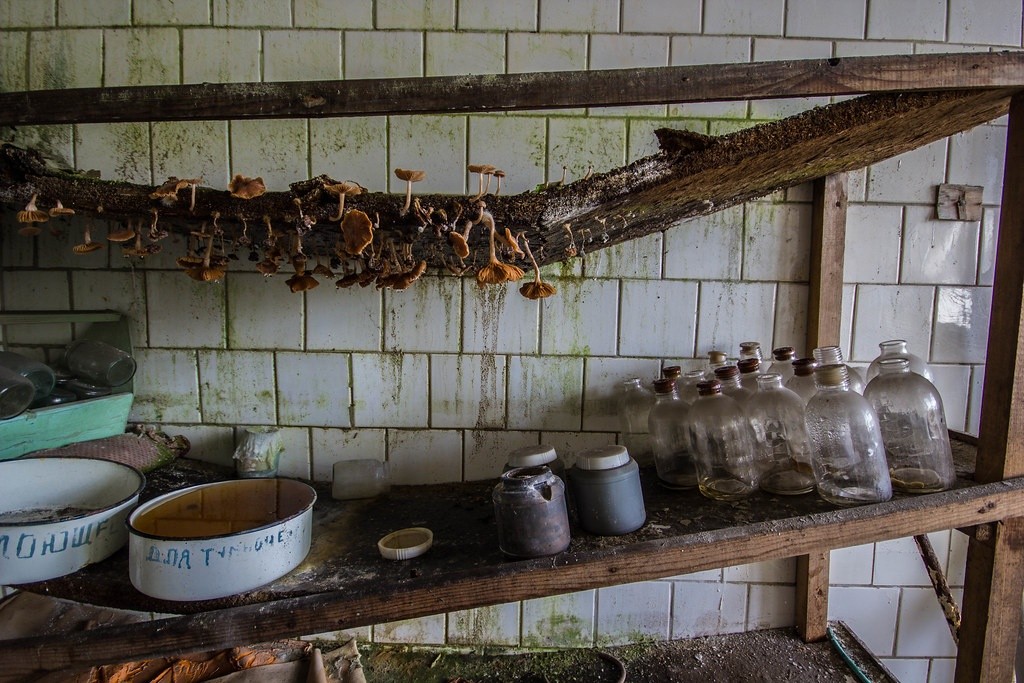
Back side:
[13,162,557,301]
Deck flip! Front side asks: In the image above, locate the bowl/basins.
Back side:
[125,475,317,602]
[0,459,147,586]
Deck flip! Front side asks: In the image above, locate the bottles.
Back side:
[616,341,959,507]
[0,336,137,420]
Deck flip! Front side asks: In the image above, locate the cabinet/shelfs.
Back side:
[0,49,1023,683]
